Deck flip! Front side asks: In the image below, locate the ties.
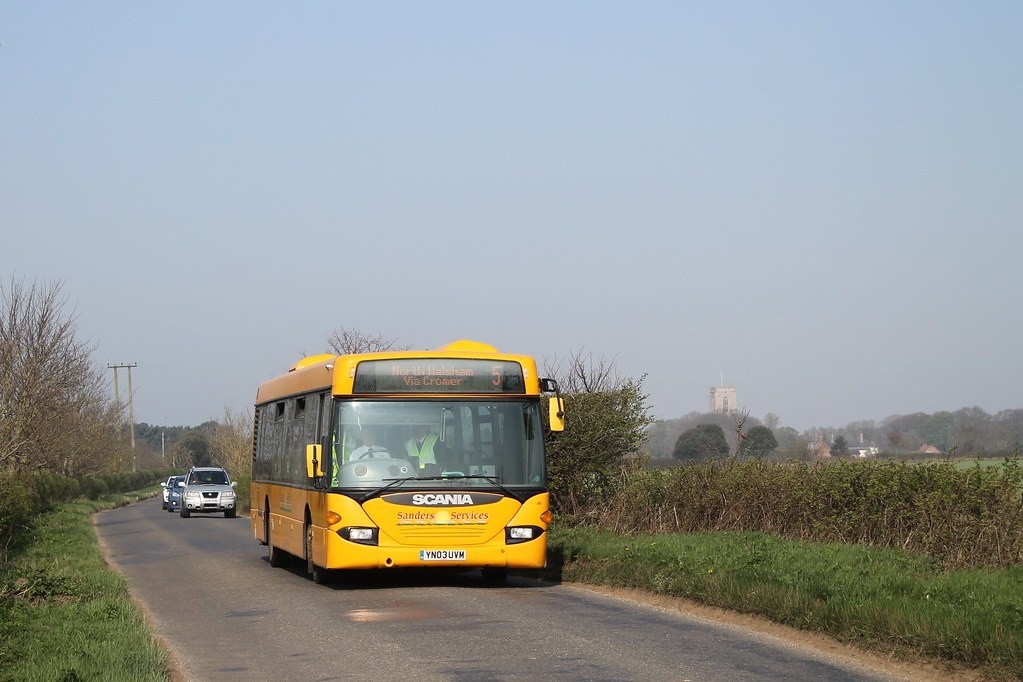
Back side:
[367,448,374,458]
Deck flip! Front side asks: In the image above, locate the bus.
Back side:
[251,339,565,586]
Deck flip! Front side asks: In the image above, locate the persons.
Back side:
[402,418,448,480]
[347,427,391,463]
[177,480,183,486]
[189,474,200,484]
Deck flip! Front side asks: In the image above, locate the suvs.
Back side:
[177,465,239,518]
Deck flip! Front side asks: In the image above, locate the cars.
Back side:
[165,476,186,512]
[160,476,177,510]
